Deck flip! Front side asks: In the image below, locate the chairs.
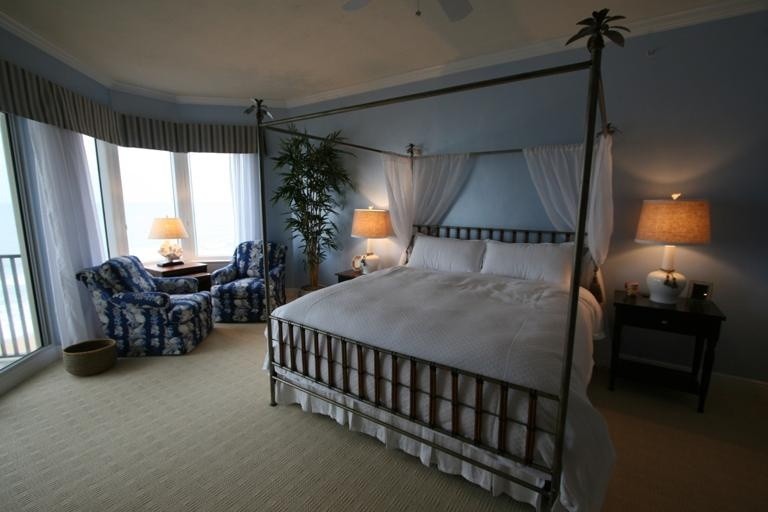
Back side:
[76,255,213,356]
[211,240,288,322]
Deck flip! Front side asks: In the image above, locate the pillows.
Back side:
[483,239,575,286]
[405,232,485,273]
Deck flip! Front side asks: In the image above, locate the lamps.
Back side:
[634,199,712,304]
[147,218,189,268]
[350,209,393,275]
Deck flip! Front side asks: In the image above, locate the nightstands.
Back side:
[334,270,362,283]
[607,289,728,414]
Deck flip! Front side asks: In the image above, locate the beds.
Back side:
[243,39,610,512]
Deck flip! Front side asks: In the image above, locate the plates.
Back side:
[351,254,363,272]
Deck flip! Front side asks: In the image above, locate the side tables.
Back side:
[146,261,208,277]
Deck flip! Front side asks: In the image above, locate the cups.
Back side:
[624,281,639,296]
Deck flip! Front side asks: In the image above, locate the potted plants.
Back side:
[270,121,358,297]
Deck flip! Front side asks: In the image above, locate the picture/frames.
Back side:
[687,280,713,304]
